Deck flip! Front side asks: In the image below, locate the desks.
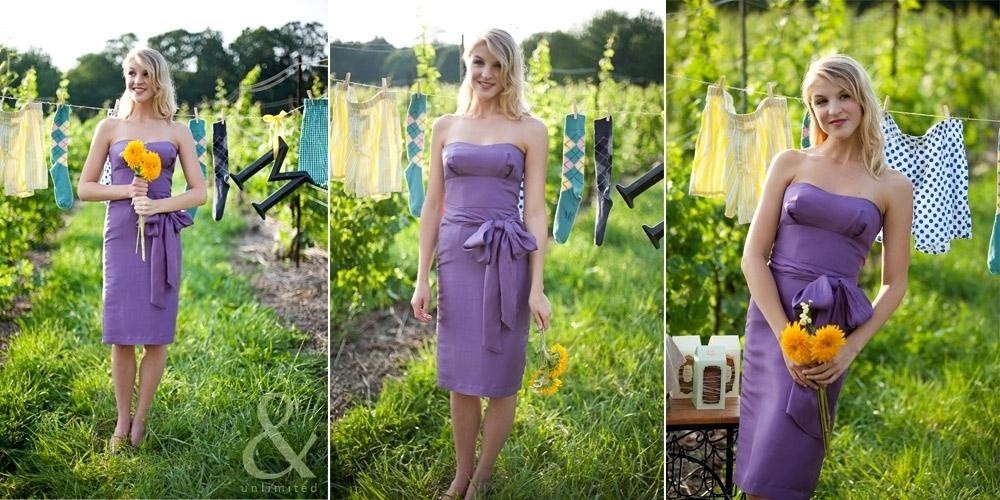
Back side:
[667,394,747,500]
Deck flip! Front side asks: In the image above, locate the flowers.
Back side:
[123,140,162,260]
[780,299,849,462]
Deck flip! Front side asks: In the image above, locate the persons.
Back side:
[411,29,552,499]
[77,45,208,451]
[721,54,913,500]
